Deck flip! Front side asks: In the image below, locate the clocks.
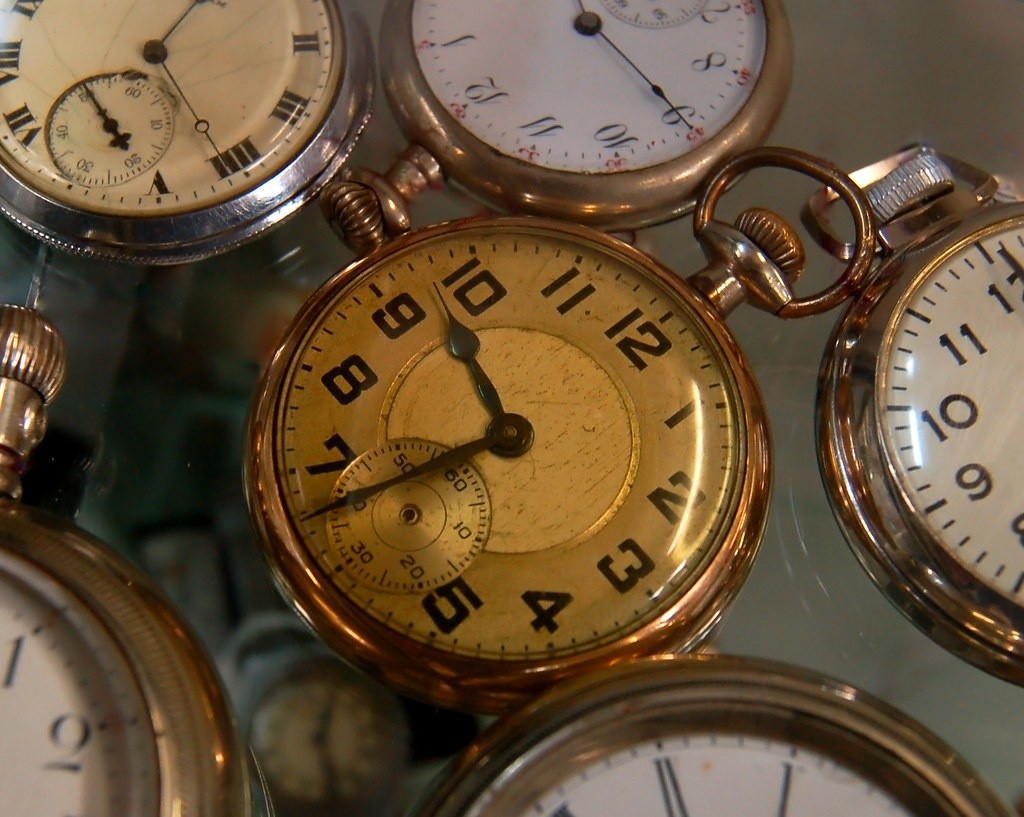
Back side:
[409,654,1016,817]
[0,0,377,268]
[243,144,876,715]
[320,0,792,254]
[800,143,1024,688]
[0,278,272,817]
